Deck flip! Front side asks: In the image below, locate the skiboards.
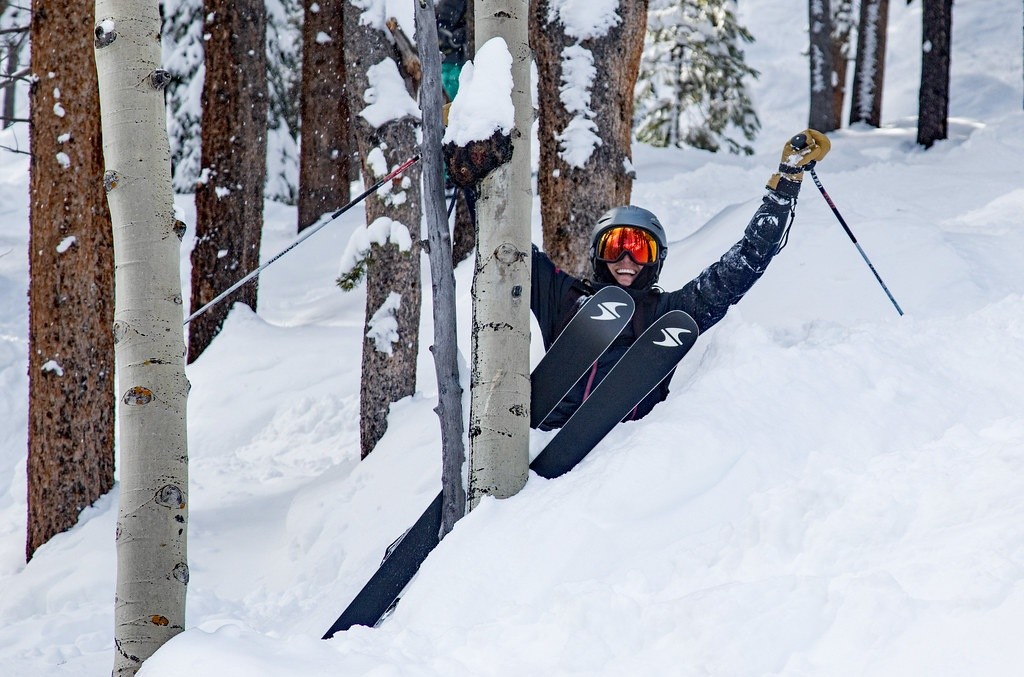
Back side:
[320,284,702,641]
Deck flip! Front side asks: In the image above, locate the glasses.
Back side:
[596,225,660,265]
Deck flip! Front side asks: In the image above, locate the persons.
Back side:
[466,126,830,432]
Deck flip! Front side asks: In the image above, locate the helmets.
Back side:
[589,205,668,275]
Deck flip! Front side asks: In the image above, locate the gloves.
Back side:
[767,129,831,197]
